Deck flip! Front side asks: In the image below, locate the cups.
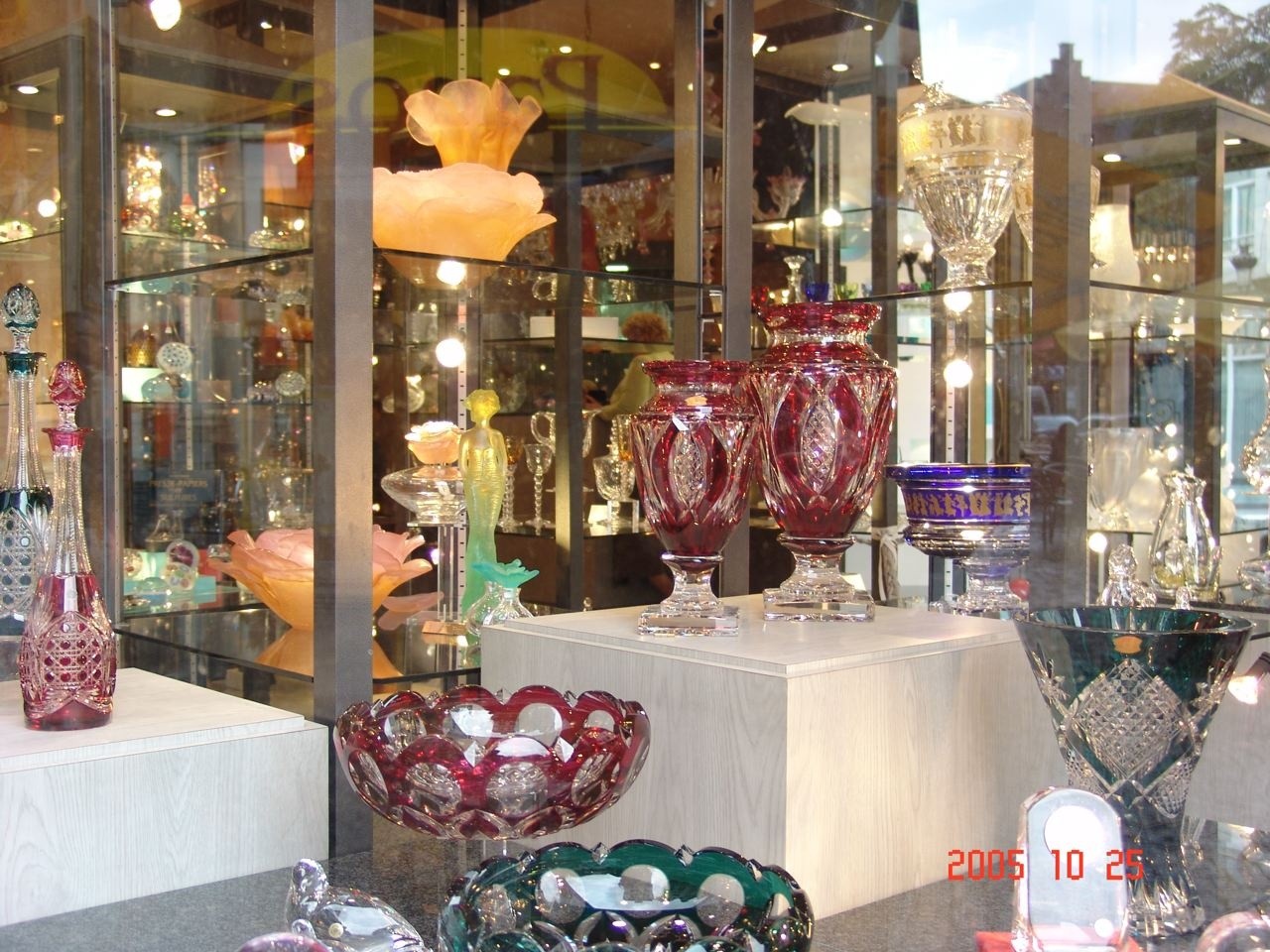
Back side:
[1180,546,1221,602]
[1090,427,1154,530]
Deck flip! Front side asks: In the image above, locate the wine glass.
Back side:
[497,435,523,528]
[884,463,1031,613]
[1013,604,1256,939]
[523,444,552,525]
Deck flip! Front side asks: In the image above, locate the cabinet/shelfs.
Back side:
[717,0,1269,610]
[77,0,729,858]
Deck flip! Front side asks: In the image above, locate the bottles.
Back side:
[1149,471,1221,603]
[629,302,898,635]
[465,558,540,637]
[898,57,1102,290]
[0,282,53,642]
[17,358,118,731]
[1239,365,1270,494]
